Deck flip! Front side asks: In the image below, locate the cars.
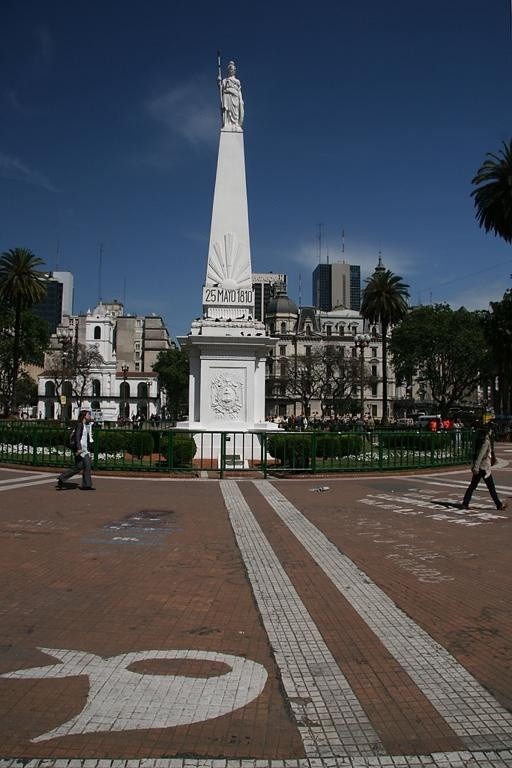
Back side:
[4,404,38,428]
[397,408,495,430]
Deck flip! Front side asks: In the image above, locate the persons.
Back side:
[217,59,243,129]
[266,411,511,433]
[461,424,510,510]
[56,409,97,491]
[17,408,189,431]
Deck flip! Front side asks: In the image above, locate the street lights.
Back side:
[57,330,73,420]
[354,333,370,420]
[121,362,129,421]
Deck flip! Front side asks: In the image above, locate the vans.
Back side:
[89,409,104,428]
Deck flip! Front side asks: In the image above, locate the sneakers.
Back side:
[497,503,509,510]
[56,476,63,489]
[81,485,95,491]
[459,502,473,510]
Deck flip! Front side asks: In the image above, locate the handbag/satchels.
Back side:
[65,422,81,452]
